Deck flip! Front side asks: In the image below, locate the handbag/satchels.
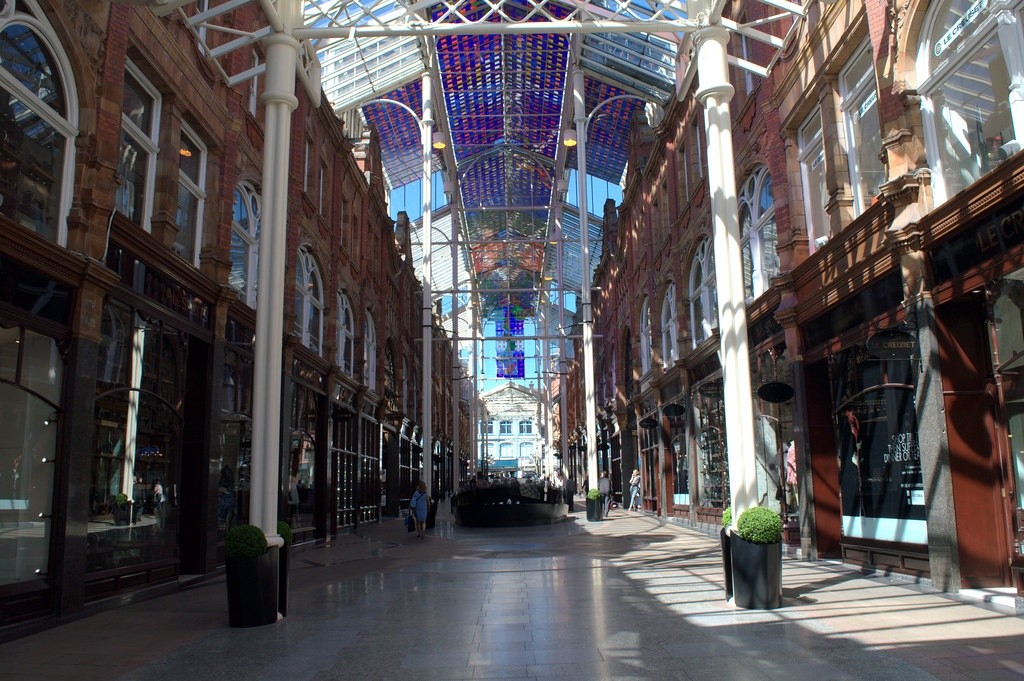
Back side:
[404,510,416,532]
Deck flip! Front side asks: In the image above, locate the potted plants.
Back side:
[730,507,784,610]
[277,520,293,617]
[223,524,278,628]
[111,493,130,525]
[586,489,603,522]
[720,507,734,602]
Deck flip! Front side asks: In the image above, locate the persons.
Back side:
[582,469,612,522]
[460,470,575,515]
[628,468,642,512]
[410,481,431,539]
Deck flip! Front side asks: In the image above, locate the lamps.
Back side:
[443,182,453,194]
[545,271,553,281]
[557,178,568,191]
[432,131,446,150]
[564,129,577,146]
